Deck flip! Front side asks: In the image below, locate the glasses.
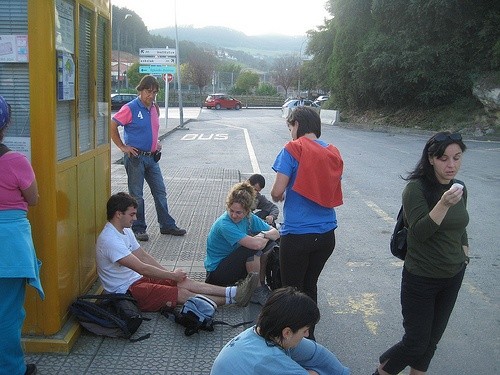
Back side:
[431,132,462,145]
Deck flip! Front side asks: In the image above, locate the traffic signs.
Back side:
[138,65,177,75]
[139,56,177,66]
[139,48,176,57]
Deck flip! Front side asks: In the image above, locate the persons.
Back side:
[204,181,280,306]
[269,106,344,344]
[0,95,46,375]
[247,174,279,228]
[369,130,470,375]
[210,287,353,375]
[110,75,187,242]
[95,192,259,312]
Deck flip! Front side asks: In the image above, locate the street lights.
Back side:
[117,14,133,94]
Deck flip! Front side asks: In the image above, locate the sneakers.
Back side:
[233,272,258,307]
[135,231,148,241]
[161,226,186,235]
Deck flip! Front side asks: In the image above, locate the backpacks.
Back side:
[259,242,284,293]
[390,179,457,259]
[68,292,152,341]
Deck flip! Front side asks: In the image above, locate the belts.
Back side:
[134,149,157,156]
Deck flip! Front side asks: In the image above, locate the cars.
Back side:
[205,94,243,111]
[280,96,329,112]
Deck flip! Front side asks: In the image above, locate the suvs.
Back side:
[110,94,138,110]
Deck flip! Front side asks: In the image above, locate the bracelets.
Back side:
[261,230,267,238]
[466,256,469,263]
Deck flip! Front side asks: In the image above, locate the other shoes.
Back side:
[25,364,36,375]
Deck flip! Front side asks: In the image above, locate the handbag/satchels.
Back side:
[181,294,218,336]
